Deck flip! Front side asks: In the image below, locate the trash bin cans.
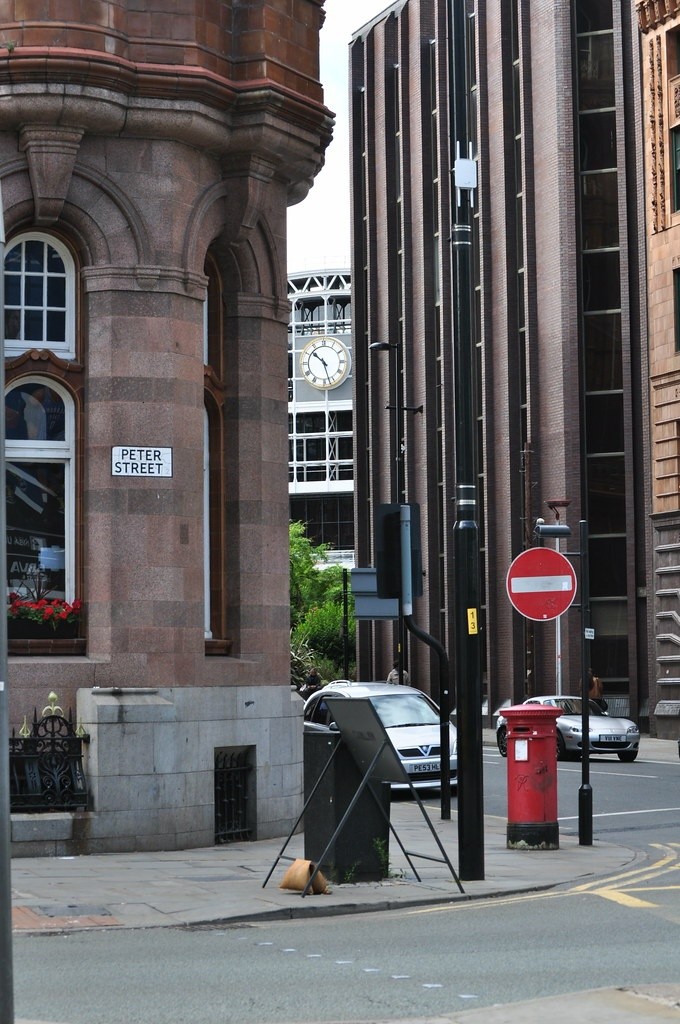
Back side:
[303,731,390,883]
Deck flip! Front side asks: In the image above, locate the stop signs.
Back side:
[504,545,577,622]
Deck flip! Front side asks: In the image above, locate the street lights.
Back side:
[533,500,575,696]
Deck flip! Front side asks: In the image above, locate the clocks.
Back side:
[299,337,351,390]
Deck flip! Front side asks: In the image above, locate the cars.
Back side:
[303,678,458,797]
[496,694,641,762]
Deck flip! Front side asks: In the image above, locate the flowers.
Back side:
[9,593,48,622]
[39,599,83,629]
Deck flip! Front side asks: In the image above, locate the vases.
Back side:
[48,621,78,640]
[11,619,38,639]
